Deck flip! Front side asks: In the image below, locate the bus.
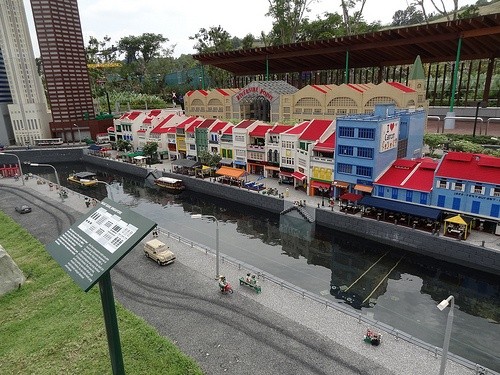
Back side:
[34,138,64,147]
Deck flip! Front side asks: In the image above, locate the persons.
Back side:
[84,198,97,208]
[153,227,158,236]
[218,275,228,291]
[246,273,257,288]
[49,183,53,191]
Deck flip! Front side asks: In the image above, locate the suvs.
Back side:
[143,238,177,267]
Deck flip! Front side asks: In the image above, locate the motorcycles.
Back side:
[220,283,234,295]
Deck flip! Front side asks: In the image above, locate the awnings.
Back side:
[335,182,349,189]
[291,171,306,180]
[219,158,233,165]
[359,195,440,219]
[354,184,373,193]
[311,181,330,190]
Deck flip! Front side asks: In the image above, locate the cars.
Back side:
[14,204,32,214]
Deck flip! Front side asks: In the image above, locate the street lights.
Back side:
[29,163,61,189]
[191,214,222,280]
[73,124,82,144]
[0,153,24,185]
[436,294,455,375]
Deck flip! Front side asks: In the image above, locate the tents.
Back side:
[87,145,112,158]
[442,214,472,239]
[195,165,211,177]
[171,159,203,178]
[339,193,363,213]
[134,156,148,165]
[215,166,250,185]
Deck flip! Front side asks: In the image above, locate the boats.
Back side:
[154,176,184,192]
[67,170,99,190]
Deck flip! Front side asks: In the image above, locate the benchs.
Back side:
[239,277,261,293]
